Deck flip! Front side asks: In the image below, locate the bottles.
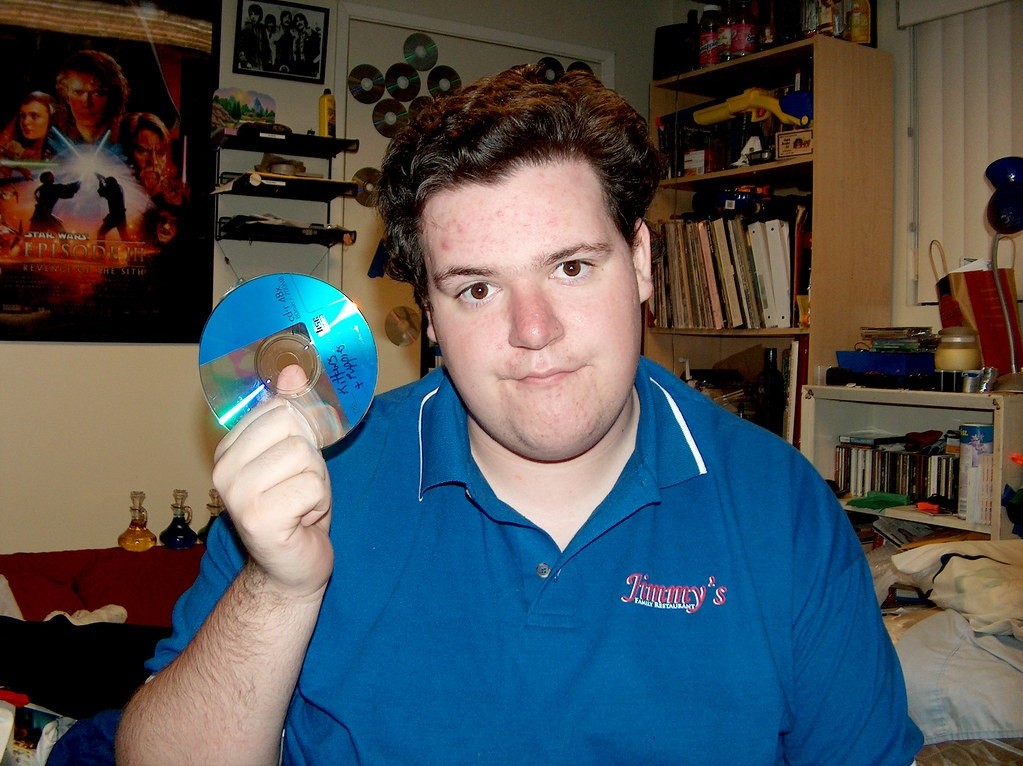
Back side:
[118,490,156,552]
[319,88,336,138]
[160,488,198,549]
[754,346,784,424]
[198,489,222,543]
[697,3,767,68]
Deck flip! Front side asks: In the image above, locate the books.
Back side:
[645,213,789,329]
[833,427,959,507]
[872,518,934,549]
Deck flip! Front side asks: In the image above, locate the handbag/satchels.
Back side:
[929,235,1023,379]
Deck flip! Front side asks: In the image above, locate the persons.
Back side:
[0,91,58,179]
[117,112,189,244]
[237,4,321,77]
[898,527,916,542]
[40,50,129,161]
[29,172,80,252]
[95,175,135,257]
[113,63,924,766]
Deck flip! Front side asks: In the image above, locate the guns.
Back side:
[656,87,815,129]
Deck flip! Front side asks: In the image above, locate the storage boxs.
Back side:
[834,350,935,389]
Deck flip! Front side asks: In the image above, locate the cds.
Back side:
[198,272,378,450]
[567,62,594,75]
[353,167,381,207]
[385,306,421,347]
[349,32,462,139]
[536,57,564,85]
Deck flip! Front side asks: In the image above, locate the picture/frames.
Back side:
[232,0,330,85]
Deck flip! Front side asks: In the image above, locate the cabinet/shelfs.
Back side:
[215,132,361,248]
[799,385,1023,540]
[644,33,897,385]
